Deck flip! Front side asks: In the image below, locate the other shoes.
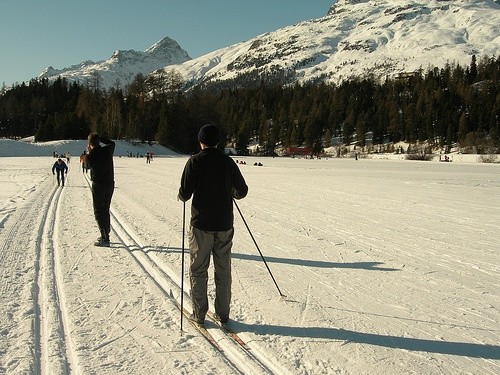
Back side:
[94,234,110,247]
[213,313,229,323]
[189,314,204,324]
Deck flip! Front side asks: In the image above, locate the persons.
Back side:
[177,123,248,326]
[127,150,155,164]
[84,132,116,246]
[52,158,68,188]
[80,150,88,174]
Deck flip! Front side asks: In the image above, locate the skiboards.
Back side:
[170,289,252,351]
